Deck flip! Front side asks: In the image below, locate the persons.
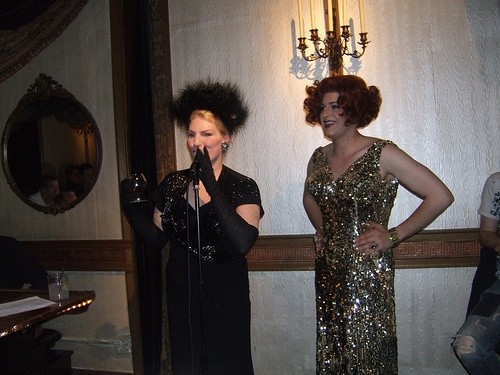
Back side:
[23,161,95,208]
[118,78,265,375]
[452,172,500,375]
[302,74,454,375]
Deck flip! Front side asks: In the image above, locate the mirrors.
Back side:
[0,73,103,216]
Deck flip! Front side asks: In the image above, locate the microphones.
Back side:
[190,155,200,178]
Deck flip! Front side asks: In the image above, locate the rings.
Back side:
[371,242,376,249]
[314,235,319,242]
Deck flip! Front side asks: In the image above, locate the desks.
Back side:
[0,288,95,375]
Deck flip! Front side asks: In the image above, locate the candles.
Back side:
[327,0,333,31]
[342,0,348,25]
[310,0,316,29]
[297,0,305,38]
[359,0,366,33]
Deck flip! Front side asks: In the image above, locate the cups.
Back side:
[46,270,69,300]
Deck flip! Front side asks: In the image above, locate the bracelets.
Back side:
[316,226,324,230]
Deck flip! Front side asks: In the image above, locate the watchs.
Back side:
[388,227,400,249]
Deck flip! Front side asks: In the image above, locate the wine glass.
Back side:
[127,173,148,204]
